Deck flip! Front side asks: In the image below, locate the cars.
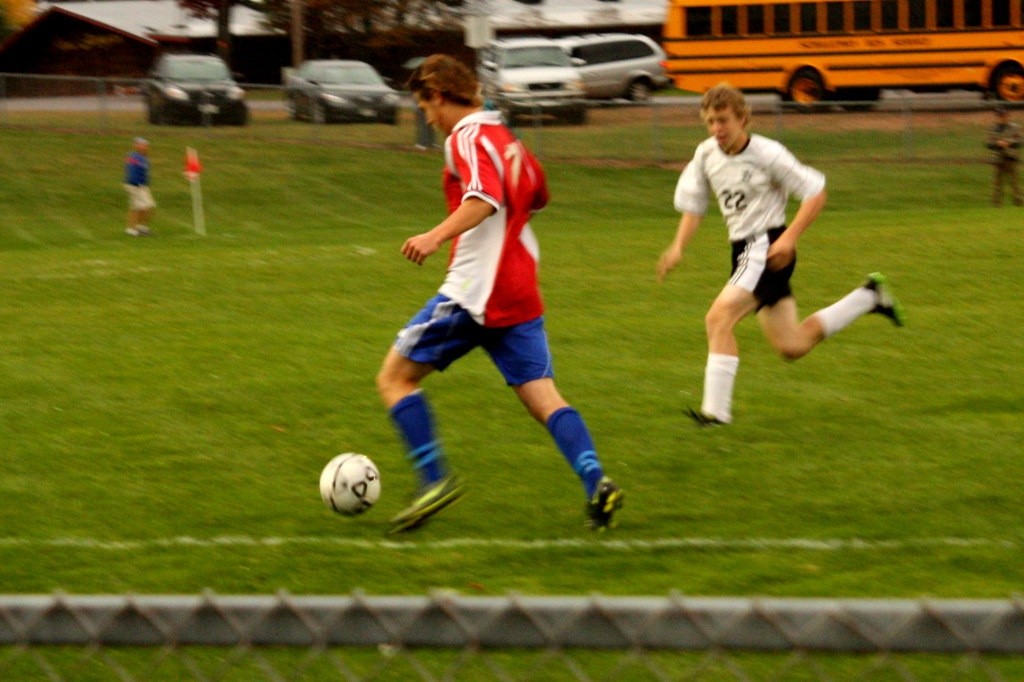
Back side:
[562,32,674,101]
[282,61,400,124]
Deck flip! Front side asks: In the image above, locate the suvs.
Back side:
[476,39,585,124]
[142,51,249,125]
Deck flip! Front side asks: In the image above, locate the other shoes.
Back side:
[862,271,907,327]
[386,473,470,535]
[126,229,138,236]
[136,225,150,234]
[682,406,722,426]
[584,475,625,532]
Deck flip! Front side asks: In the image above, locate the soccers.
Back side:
[320,452,381,515]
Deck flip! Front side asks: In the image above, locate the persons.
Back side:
[375,54,626,537]
[983,105,1024,209]
[123,137,156,238]
[653,82,908,429]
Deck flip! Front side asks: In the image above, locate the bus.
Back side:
[657,0,1024,104]
